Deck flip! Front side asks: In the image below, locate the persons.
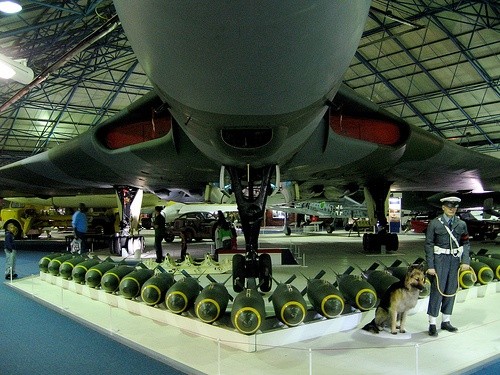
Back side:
[180,231,187,262]
[151,206,165,264]
[4,223,18,279]
[212,221,233,262]
[72,203,88,254]
[425,197,470,337]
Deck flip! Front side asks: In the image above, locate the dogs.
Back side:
[361,262,427,334]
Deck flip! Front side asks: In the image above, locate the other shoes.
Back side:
[5,274,17,279]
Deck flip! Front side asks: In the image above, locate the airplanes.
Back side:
[0,0,500,292]
[264,202,354,236]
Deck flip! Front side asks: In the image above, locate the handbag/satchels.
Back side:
[70,238,82,254]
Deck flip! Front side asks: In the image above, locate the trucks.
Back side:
[1,193,122,240]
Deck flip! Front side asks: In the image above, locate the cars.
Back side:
[166,212,234,241]
[407,216,431,235]
[457,211,500,239]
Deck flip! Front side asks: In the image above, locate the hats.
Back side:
[440,196,462,207]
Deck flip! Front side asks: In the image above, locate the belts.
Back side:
[433,245,458,255]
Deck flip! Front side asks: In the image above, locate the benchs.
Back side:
[214,248,299,265]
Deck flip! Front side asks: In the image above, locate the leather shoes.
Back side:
[441,321,458,331]
[428,324,438,336]
